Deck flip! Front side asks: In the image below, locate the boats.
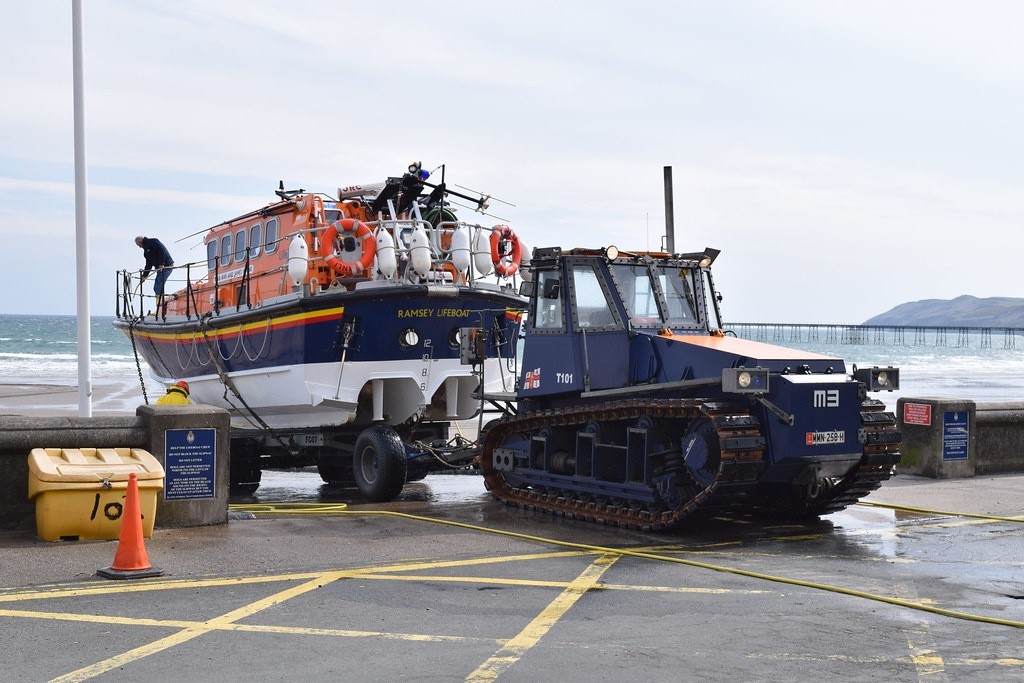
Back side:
[109,165,532,435]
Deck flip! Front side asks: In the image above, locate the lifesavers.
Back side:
[319,219,378,274]
[492,224,522,276]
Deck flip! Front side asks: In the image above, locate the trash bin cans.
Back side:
[28,448,166,542]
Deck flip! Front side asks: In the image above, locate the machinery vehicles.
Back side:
[464,164,907,534]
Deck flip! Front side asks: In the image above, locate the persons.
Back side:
[134,236,174,295]
[156,381,192,405]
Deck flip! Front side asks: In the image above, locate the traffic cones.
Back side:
[94,469,164,580]
